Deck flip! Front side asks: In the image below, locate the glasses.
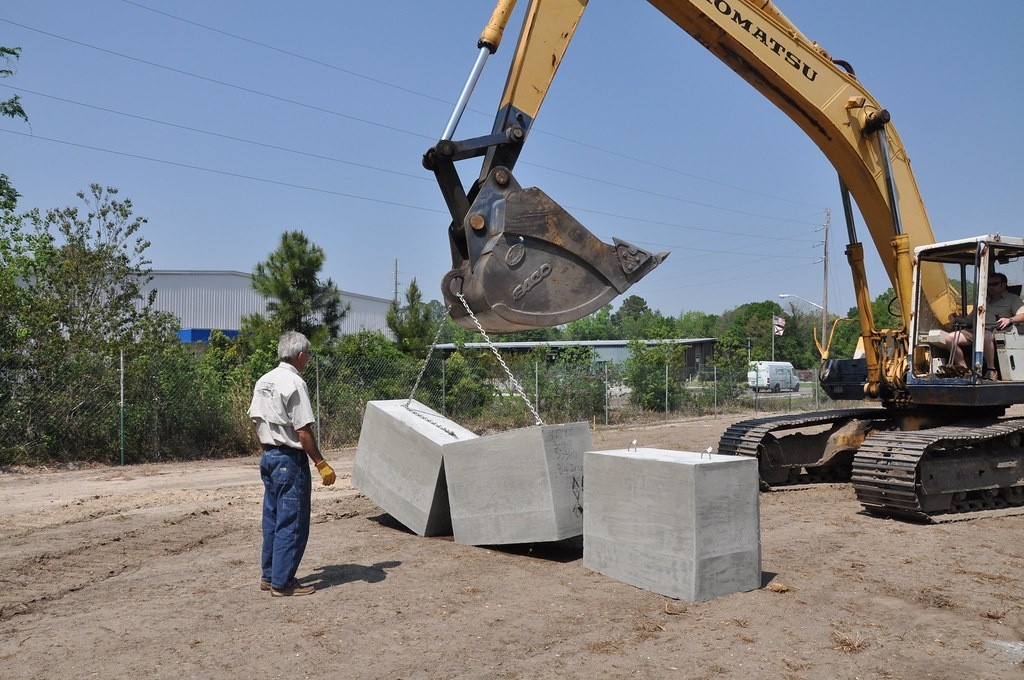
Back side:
[986,280,1004,286]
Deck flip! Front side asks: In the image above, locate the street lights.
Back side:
[779,294,827,373]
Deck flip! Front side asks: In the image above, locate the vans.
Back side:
[748,359,800,393]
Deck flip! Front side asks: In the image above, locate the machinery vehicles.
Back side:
[418,0,1024,524]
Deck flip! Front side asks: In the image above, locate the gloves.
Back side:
[314,458,337,486]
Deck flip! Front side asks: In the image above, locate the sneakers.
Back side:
[260,578,298,591]
[957,368,972,379]
[983,368,999,382]
[269,583,316,597]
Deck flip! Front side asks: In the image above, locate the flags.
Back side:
[774,326,786,336]
[773,315,786,326]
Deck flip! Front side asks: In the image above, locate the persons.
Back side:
[246,329,339,599]
[936,271,1024,380]
